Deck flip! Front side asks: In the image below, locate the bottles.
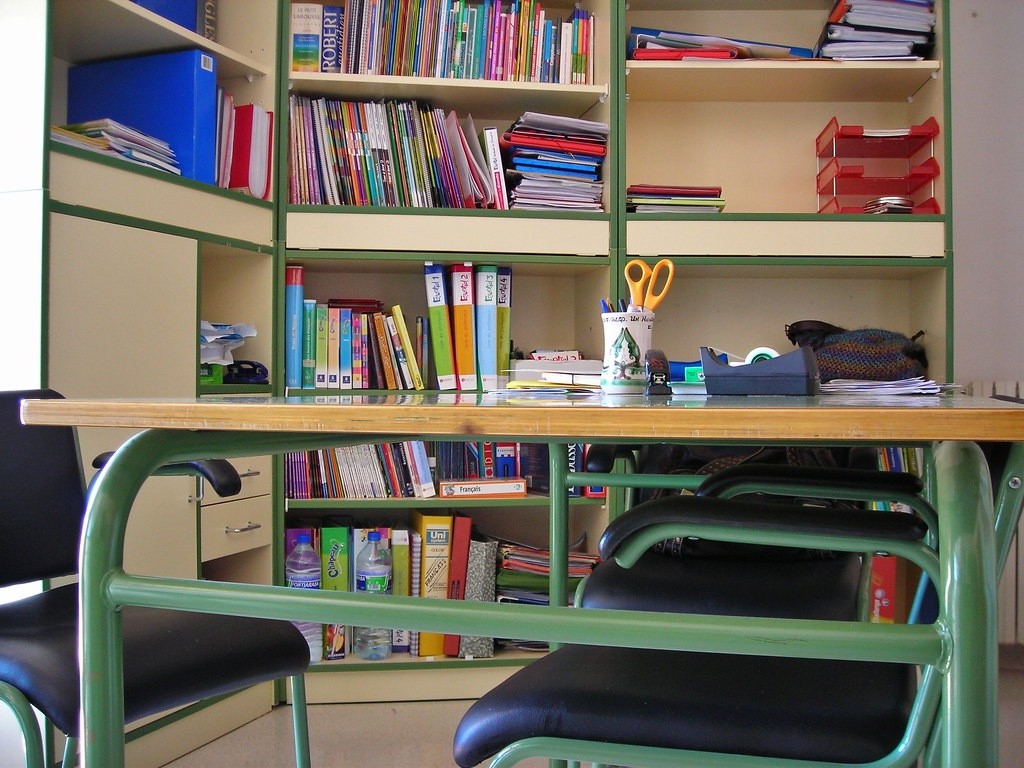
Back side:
[285,534,323,664]
[353,532,392,660]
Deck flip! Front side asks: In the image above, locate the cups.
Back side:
[600,312,655,395]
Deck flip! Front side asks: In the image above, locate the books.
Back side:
[626,183,726,214]
[289,0,594,86]
[280,437,606,663]
[68,0,274,203]
[284,262,603,389]
[287,93,610,214]
[866,447,924,624]
[627,0,936,62]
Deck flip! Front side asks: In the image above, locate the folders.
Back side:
[628,1,937,61]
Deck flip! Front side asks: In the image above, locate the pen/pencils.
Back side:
[600,297,627,313]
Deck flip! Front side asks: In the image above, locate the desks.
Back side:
[18,392,1024,768]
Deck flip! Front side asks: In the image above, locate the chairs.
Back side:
[451,394,1024,768]
[0,388,313,768]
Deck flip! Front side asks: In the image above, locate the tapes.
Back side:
[744,346,781,365]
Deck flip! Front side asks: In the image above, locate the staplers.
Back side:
[643,350,672,396]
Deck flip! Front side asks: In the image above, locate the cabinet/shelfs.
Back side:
[0,0,283,768]
[277,0,618,705]
[618,0,951,278]
[816,116,941,214]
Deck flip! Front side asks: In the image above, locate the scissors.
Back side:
[623,258,675,313]
